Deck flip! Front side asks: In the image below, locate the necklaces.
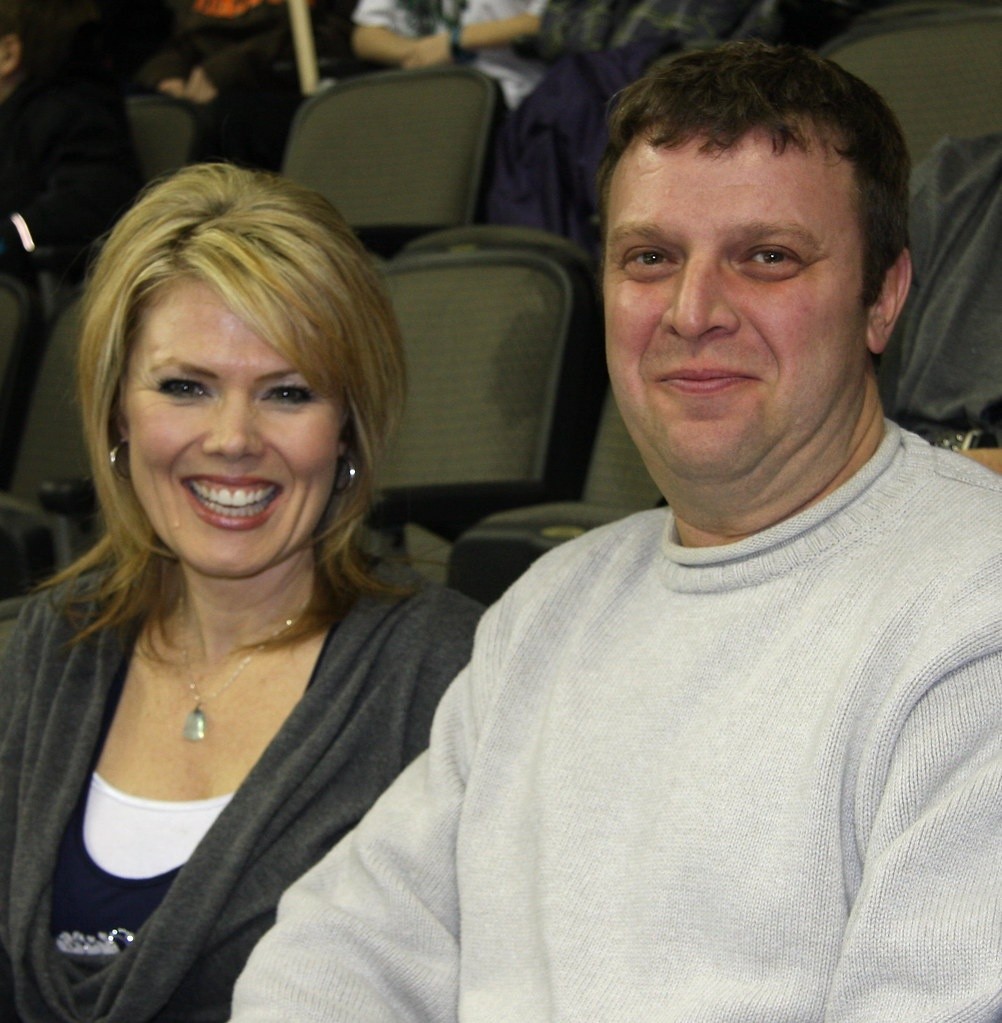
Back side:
[177,595,297,741]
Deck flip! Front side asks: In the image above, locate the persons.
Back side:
[0,162,481,1023]
[0,0,771,278]
[231,37,1002,1023]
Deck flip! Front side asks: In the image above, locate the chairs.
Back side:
[283,66,500,230]
[823,6,1002,157]
[11,286,90,506]
[379,249,573,506]
